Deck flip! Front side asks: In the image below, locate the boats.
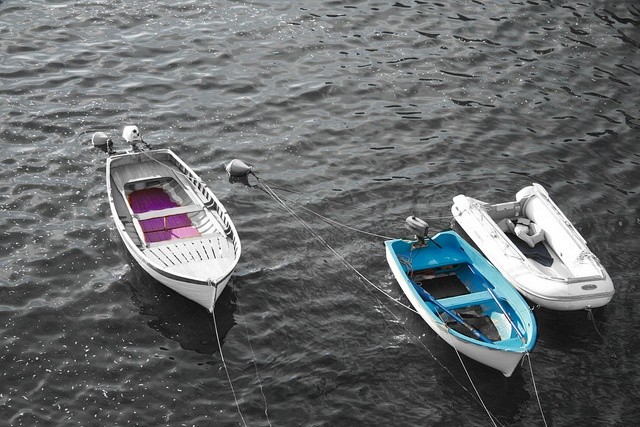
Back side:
[451,182,615,311]
[384,216,538,378]
[106,149,241,313]
